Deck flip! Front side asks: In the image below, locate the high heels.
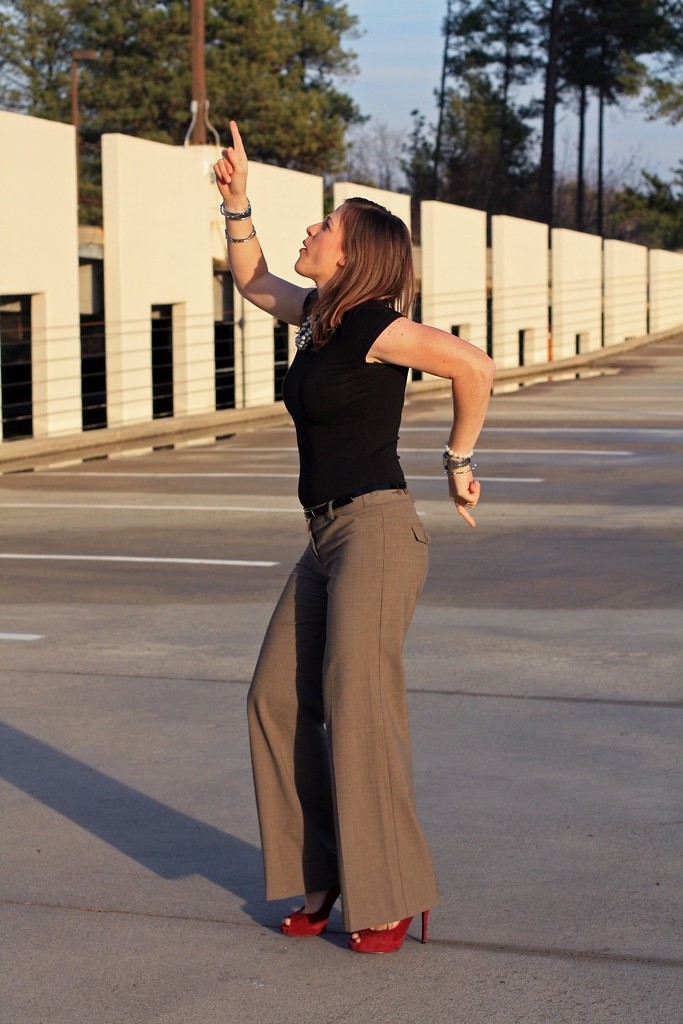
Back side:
[348,910,429,953]
[279,883,341,937]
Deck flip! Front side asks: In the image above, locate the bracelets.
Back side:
[224,224,256,244]
[444,464,478,475]
[444,442,474,459]
[220,198,251,220]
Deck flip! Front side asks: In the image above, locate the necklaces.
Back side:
[294,316,313,351]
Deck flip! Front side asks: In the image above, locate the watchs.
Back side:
[442,452,470,470]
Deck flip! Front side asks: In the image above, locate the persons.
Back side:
[212,121,496,952]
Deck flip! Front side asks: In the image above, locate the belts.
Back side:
[304,483,406,519]
